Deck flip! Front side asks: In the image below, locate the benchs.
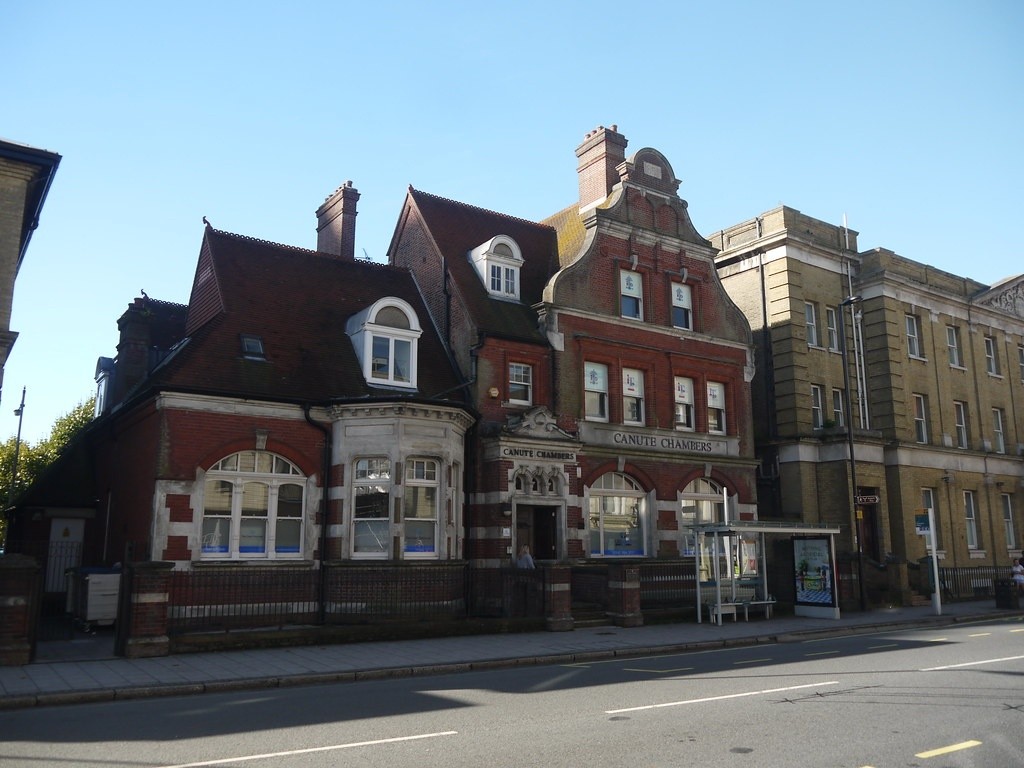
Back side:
[711,600,778,625]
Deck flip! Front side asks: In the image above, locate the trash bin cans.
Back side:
[65,568,79,612]
[995,579,1020,608]
[76,567,122,633]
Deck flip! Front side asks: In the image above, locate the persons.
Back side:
[1011,549,1024,594]
[800,563,827,592]
[516,542,536,569]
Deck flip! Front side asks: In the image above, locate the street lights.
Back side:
[5,386,29,505]
[837,296,866,599]
